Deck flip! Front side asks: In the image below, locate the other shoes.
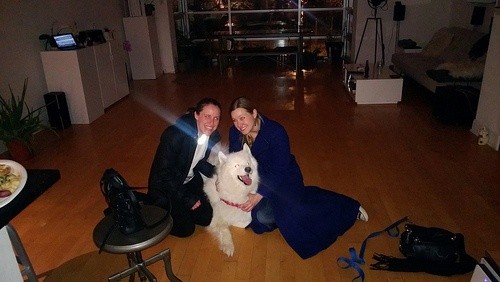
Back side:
[358,206,368,222]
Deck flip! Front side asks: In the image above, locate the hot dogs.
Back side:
[0,190,12,197]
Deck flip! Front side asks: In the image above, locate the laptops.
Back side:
[51,33,84,50]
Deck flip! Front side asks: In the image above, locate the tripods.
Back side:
[354,0,385,65]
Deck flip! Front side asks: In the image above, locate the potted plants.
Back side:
[175,30,189,73]
[0,78,60,162]
[144,0,155,16]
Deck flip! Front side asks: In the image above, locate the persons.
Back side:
[133,98,219,237]
[229,98,369,260]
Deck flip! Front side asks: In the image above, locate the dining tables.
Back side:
[0,169,60,282]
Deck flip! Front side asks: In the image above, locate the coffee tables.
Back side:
[342,64,403,104]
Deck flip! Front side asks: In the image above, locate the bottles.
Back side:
[378,60,382,77]
[365,60,369,79]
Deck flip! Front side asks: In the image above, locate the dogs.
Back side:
[203,143,260,257]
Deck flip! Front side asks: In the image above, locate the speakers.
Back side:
[44,92,71,131]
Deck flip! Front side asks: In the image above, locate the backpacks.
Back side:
[370,224,479,277]
[97,167,147,235]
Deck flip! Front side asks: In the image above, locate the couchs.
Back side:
[391,26,490,105]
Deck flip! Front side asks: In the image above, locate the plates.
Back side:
[0,159,27,208]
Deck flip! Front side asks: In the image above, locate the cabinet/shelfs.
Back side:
[40,37,130,124]
[219,33,300,78]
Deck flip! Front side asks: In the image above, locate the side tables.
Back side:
[122,16,163,81]
[400,48,423,53]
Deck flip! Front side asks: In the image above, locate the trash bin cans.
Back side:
[43,92,72,131]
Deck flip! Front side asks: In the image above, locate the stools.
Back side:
[93,205,184,282]
